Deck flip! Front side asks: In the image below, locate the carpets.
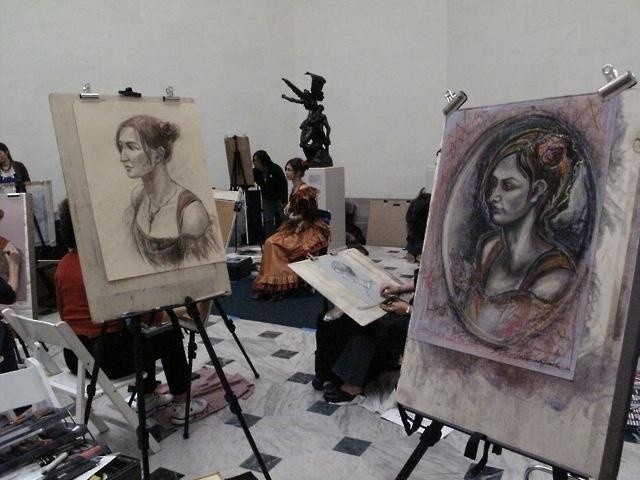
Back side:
[30,310,640,480]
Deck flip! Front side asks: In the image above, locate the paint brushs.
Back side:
[3,250,10,255]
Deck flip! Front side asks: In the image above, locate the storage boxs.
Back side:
[227,257,253,279]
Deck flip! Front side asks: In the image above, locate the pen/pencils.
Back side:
[42,450,71,475]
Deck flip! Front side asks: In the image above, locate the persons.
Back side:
[0,143,34,202]
[0,241,25,371]
[110,112,227,272]
[246,156,328,302]
[252,149,289,210]
[322,188,431,405]
[51,194,213,421]
[461,130,582,353]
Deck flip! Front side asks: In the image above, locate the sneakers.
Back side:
[312,378,363,403]
[136,392,208,424]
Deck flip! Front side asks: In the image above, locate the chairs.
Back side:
[0,358,61,432]
[0,306,164,455]
